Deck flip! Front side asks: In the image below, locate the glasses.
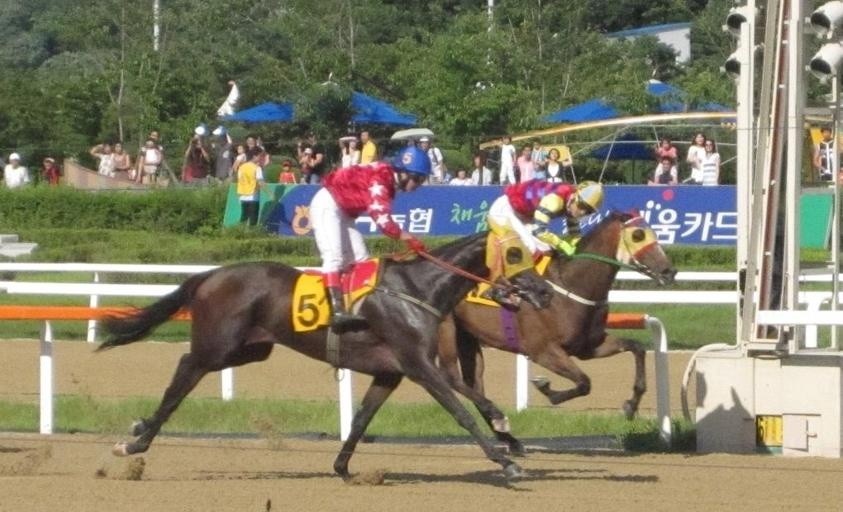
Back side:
[704,144,713,147]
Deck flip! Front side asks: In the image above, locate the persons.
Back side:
[654,137,678,182]
[813,126,835,180]
[88,131,165,187]
[182,132,444,186]
[43,157,59,185]
[648,155,677,185]
[235,146,268,227]
[441,134,573,186]
[4,152,31,187]
[701,138,720,185]
[687,131,708,184]
[308,145,434,331]
[487,180,605,312]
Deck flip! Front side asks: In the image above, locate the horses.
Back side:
[378,200,678,459]
[88,194,535,494]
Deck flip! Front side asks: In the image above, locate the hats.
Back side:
[282,160,291,167]
[419,137,430,142]
[304,148,314,154]
[305,130,314,136]
[7,153,21,161]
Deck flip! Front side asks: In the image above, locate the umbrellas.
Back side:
[584,133,657,183]
[532,79,735,122]
[224,91,417,129]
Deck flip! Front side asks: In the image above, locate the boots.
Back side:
[328,286,368,336]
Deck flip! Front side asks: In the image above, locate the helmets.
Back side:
[393,147,432,176]
[576,179,603,212]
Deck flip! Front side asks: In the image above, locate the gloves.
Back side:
[406,231,426,256]
[556,236,576,258]
